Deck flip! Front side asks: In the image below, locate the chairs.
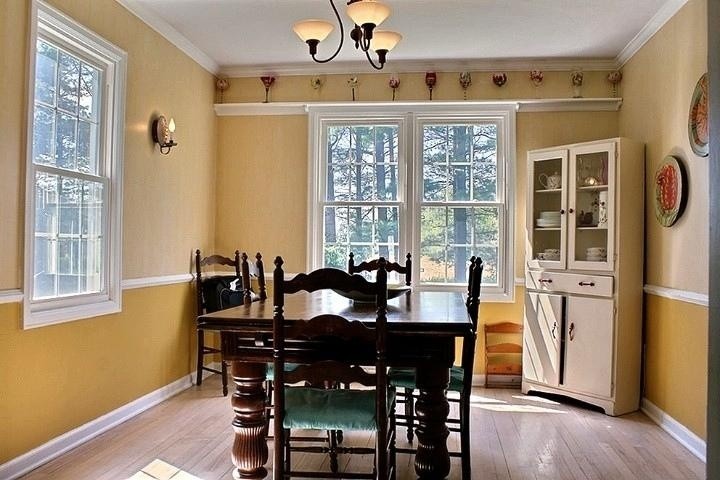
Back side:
[344,253,414,443]
[388,256,484,480]
[273,256,396,480]
[242,252,343,472]
[195,249,242,397]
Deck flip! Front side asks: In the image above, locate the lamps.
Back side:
[155,115,177,155]
[290,0,402,70]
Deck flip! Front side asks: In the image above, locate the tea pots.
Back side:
[539,171,561,188]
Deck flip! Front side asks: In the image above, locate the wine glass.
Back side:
[459,71,472,100]
[425,72,436,100]
[348,73,359,101]
[260,77,276,103]
[311,76,322,101]
[389,72,400,101]
[529,71,544,98]
[217,79,229,103]
[608,71,622,98]
[492,72,507,99]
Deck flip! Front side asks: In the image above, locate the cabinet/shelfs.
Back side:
[484,322,524,388]
[521,137,645,417]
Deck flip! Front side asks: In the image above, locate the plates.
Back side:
[653,155,683,228]
[536,211,561,227]
[331,283,412,304]
[688,72,709,157]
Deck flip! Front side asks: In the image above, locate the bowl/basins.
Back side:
[584,247,607,261]
[537,253,559,260]
[544,249,560,256]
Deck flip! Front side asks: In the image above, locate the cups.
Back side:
[572,71,584,97]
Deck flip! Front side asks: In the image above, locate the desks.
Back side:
[195,289,473,480]
